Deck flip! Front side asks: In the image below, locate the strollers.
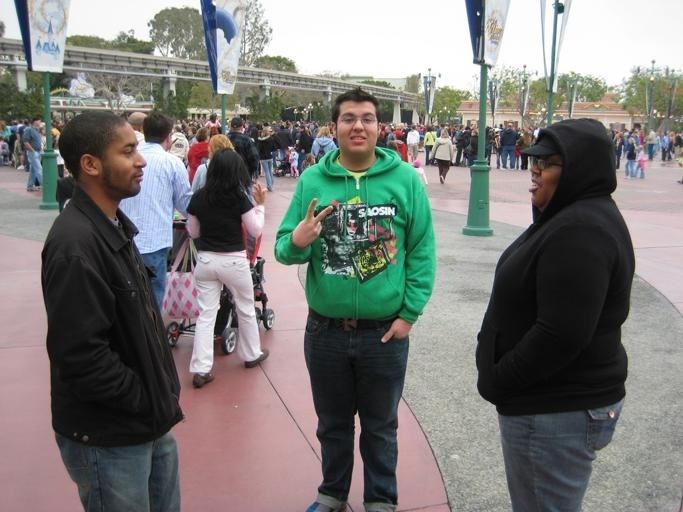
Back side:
[161,217,275,356]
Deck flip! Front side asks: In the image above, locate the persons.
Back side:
[192,134,235,194]
[446,123,478,167]
[609,127,682,179]
[272,86,436,512]
[472,119,637,512]
[184,146,270,389]
[168,114,224,185]
[431,129,453,184]
[487,123,544,170]
[1,117,65,192]
[228,117,260,182]
[243,120,339,191]
[115,113,194,314]
[376,121,445,165]
[412,160,429,185]
[128,114,146,143]
[37,111,185,512]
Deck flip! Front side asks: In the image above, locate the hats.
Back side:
[522,137,562,155]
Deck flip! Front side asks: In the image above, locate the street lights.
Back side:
[462,1,509,237]
[540,1,571,128]
[293,102,313,122]
[418,68,442,126]
[474,71,504,129]
[438,106,451,124]
[561,70,581,121]
[635,58,664,133]
[510,63,538,130]
[662,68,681,131]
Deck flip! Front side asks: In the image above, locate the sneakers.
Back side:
[245,350,269,367]
[193,373,215,388]
[305,502,338,512]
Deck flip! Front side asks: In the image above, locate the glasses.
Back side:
[530,157,563,170]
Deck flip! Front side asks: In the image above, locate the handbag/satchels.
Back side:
[160,272,203,319]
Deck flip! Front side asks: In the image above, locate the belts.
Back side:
[309,309,399,332]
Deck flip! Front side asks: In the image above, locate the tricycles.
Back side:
[274,158,292,176]
